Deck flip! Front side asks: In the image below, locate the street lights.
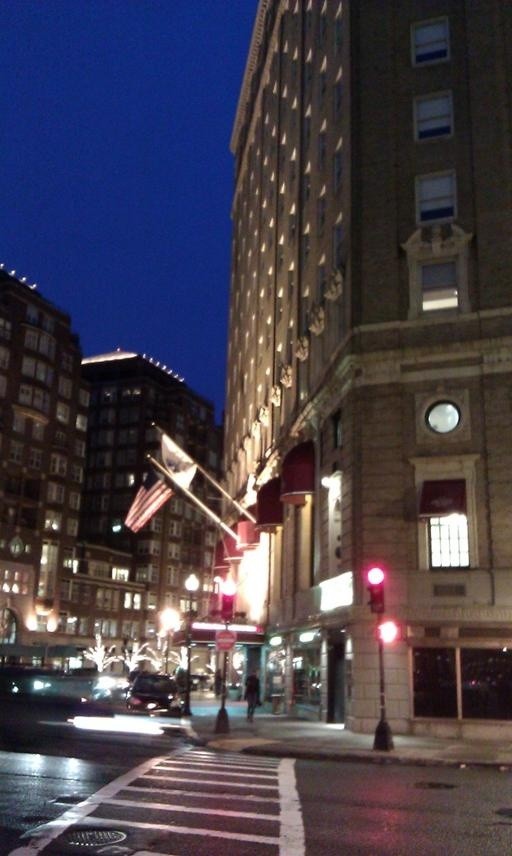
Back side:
[184,573,200,716]
[160,607,179,674]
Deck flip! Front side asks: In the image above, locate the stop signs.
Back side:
[215,631,236,650]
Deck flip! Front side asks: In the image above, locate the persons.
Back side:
[213,667,223,699]
[245,674,259,721]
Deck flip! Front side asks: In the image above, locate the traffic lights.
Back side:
[367,563,386,614]
[377,623,402,642]
[221,580,236,621]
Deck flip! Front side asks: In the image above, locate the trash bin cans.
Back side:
[271,694,285,715]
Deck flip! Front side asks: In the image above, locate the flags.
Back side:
[123,463,177,535]
[153,433,197,490]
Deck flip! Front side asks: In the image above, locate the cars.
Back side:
[1,642,215,723]
